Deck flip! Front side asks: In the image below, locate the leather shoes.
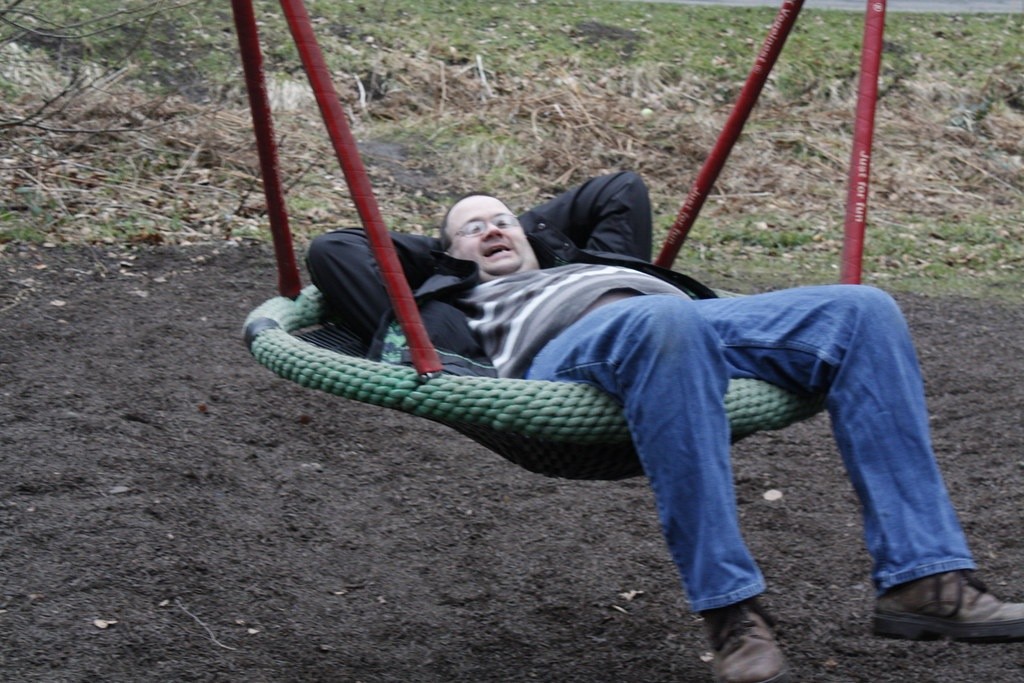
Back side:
[706,596,789,683]
[871,568,1024,642]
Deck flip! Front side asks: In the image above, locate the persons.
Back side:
[306,169,1023,683]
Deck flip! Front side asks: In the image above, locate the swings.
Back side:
[232,2,886,480]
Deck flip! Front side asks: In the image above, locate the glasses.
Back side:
[452,214,518,237]
